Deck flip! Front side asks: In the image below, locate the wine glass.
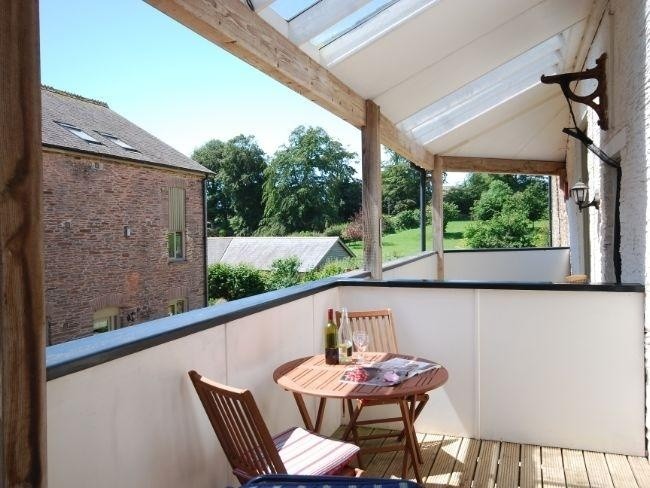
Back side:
[352,330,370,365]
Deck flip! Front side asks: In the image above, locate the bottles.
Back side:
[337,308,353,364]
[325,308,338,364]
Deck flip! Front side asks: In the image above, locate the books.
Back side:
[338,355,442,386]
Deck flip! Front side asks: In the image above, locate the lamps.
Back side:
[570,176,600,214]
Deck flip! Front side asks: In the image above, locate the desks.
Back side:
[273,351,448,486]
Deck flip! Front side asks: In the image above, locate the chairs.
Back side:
[334,308,429,467]
[187,369,360,485]
